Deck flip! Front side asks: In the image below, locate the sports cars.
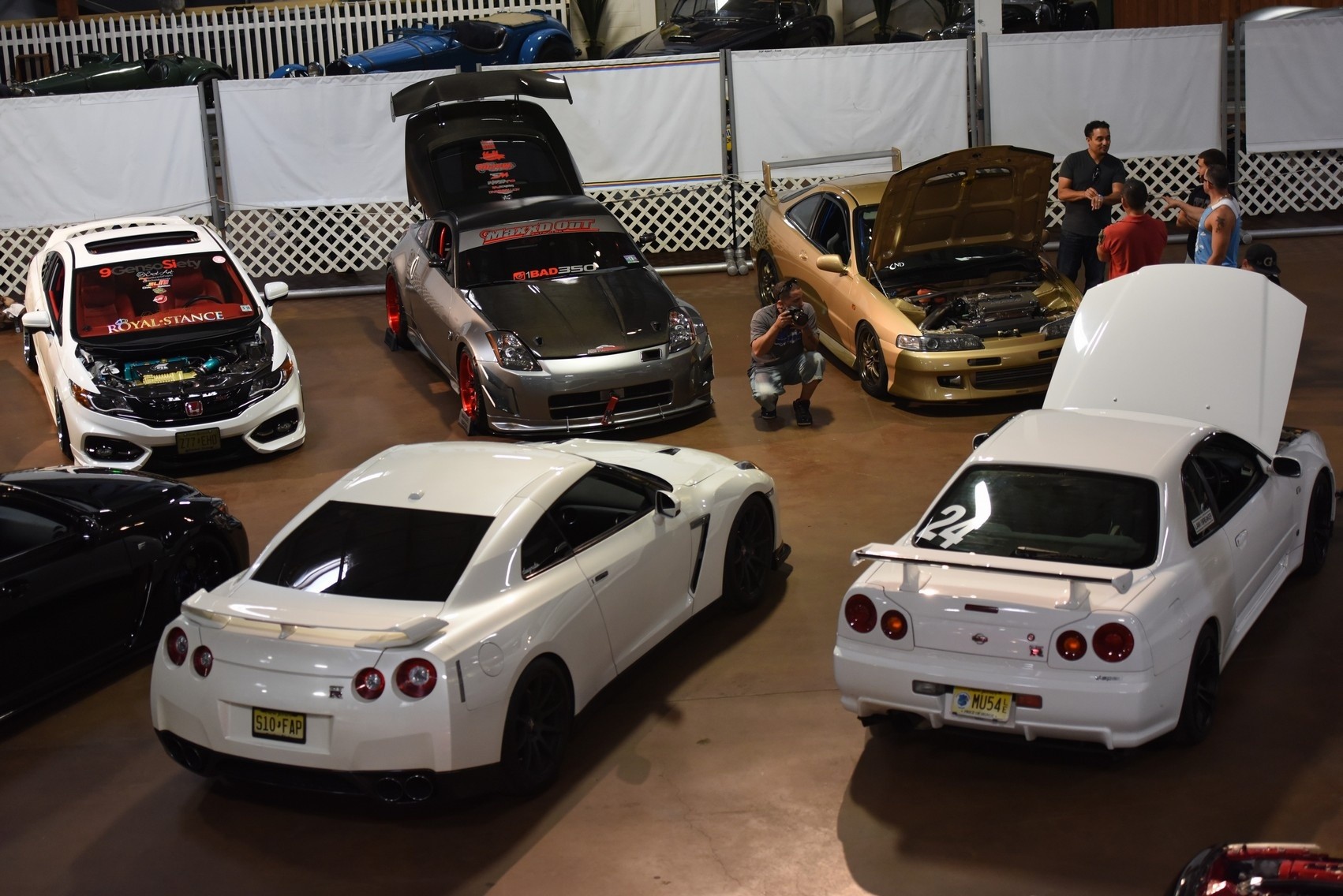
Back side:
[15,216,305,475]
[833,261,1339,750]
[146,440,783,803]
[383,67,715,438]
[0,466,251,739]
[751,146,1088,405]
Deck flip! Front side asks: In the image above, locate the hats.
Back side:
[1244,242,1282,275]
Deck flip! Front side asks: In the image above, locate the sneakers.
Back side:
[792,398,813,425]
[761,404,777,418]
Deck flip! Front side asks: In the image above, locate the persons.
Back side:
[1095,183,1170,283]
[1173,148,1228,264]
[1055,120,1131,296]
[1234,242,1281,288]
[1162,163,1243,269]
[745,277,826,426]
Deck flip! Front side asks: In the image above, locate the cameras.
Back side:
[784,305,808,326]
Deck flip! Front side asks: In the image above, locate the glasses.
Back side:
[1200,174,1214,186]
[1126,176,1136,190]
[1091,164,1100,186]
[777,278,799,300]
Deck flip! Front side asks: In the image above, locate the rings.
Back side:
[1098,200,1101,202]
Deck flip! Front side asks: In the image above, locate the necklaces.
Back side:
[1208,193,1231,207]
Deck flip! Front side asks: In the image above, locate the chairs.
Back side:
[79,276,135,329]
[155,265,225,317]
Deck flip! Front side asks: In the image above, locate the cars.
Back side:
[606,1,835,58]
[271,8,583,80]
[924,0,1101,42]
[2,46,237,109]
